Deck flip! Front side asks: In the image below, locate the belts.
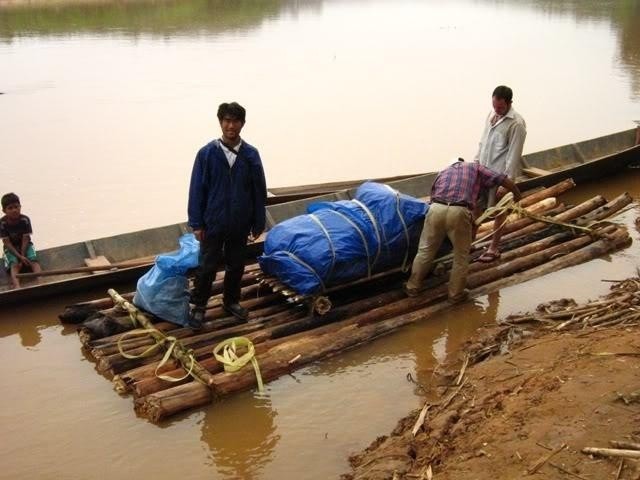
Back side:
[433,200,468,207]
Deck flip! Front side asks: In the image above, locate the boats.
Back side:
[54,179,633,427]
[1,122,640,301]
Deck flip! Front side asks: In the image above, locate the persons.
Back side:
[0,192,45,290]
[186,101,268,330]
[468,84,527,264]
[400,155,523,301]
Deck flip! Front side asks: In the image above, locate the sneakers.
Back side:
[401,281,418,298]
[189,308,205,330]
[223,302,249,319]
[447,288,470,304]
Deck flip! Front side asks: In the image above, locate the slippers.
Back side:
[479,249,500,262]
